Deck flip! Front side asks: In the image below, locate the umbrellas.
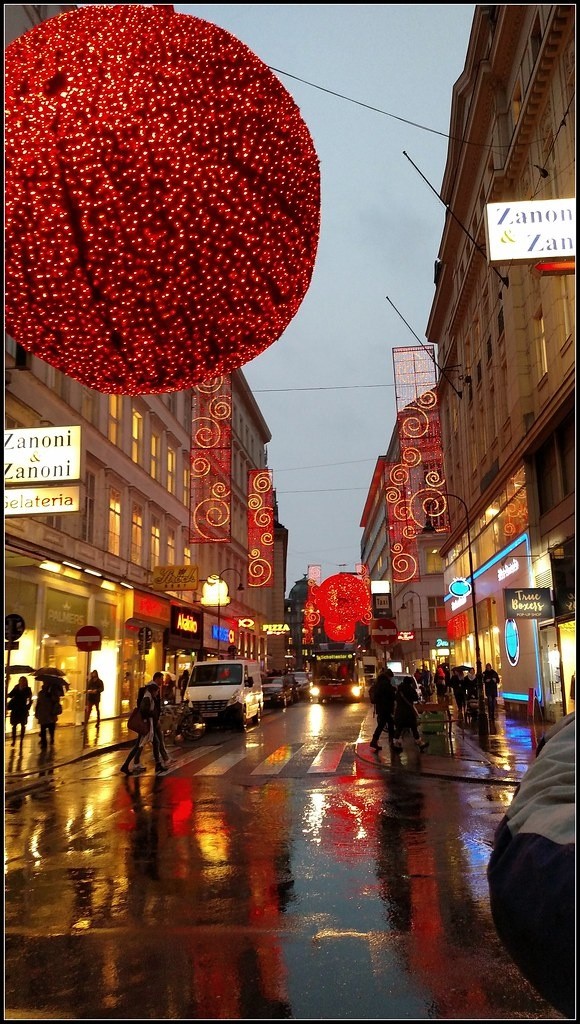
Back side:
[36,675,67,685]
[5,665,34,673]
[31,667,65,676]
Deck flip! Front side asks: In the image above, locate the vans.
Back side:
[180,659,263,733]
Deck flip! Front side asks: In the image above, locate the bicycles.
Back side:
[157,703,206,741]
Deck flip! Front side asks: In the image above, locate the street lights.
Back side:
[420,492,491,749]
[400,591,424,670]
[217,567,246,660]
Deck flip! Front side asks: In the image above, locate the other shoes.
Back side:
[370,742,382,750]
[395,745,404,751]
[121,768,132,775]
[389,743,396,750]
[51,740,54,743]
[166,760,177,765]
[155,767,168,772]
[420,743,429,752]
[41,740,47,745]
[133,767,146,772]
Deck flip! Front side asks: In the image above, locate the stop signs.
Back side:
[370,618,399,648]
[75,625,102,653]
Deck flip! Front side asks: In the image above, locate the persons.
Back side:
[485,711,576,1018]
[120,672,176,773]
[6,676,32,746]
[163,669,189,705]
[35,682,62,755]
[415,662,500,722]
[368,669,428,753]
[267,668,286,676]
[80,670,104,732]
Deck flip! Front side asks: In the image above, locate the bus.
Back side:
[307,650,366,704]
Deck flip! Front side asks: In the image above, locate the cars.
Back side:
[261,677,294,707]
[391,671,423,704]
[283,671,312,703]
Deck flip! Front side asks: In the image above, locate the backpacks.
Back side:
[137,687,145,708]
[369,685,377,704]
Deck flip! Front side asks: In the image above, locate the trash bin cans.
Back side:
[421,710,449,756]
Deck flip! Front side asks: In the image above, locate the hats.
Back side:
[385,669,394,677]
[404,677,413,684]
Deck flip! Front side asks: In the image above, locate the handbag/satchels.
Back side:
[7,699,14,710]
[127,708,150,735]
[410,704,417,716]
